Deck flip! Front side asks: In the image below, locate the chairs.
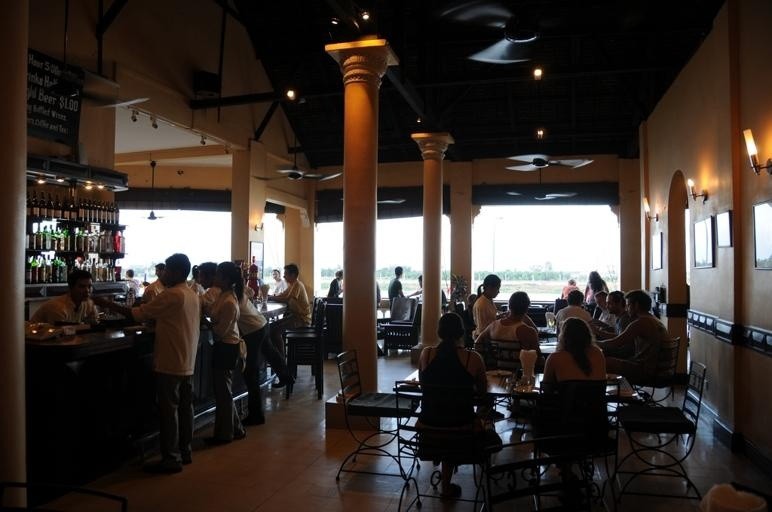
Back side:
[282,296,329,399]
[334,296,715,511]
[1,478,128,511]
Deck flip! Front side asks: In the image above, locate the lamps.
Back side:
[741,128,771,177]
[686,177,708,205]
[127,105,233,156]
[640,197,659,221]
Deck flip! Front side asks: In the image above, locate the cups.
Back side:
[260,284,271,303]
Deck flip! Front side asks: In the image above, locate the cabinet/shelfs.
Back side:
[25,159,76,287]
[75,165,133,288]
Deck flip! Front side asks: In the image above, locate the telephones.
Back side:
[25,322,63,340]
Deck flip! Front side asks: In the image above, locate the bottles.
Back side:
[26,190,127,284]
[248,255,259,295]
[520,365,536,386]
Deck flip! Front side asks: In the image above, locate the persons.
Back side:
[416,271,672,497]
[327,271,344,298]
[408,275,446,311]
[388,267,405,309]
[377,281,381,307]
[122,253,312,473]
[30,270,101,331]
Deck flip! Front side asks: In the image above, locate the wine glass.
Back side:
[548,318,555,333]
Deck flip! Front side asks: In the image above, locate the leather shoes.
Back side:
[272,377,295,387]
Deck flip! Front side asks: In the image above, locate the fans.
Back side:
[251,97,344,182]
[434,1,580,68]
[41,0,149,108]
[503,161,579,203]
[499,152,594,175]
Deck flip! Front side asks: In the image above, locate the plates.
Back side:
[26,321,59,340]
[513,385,541,393]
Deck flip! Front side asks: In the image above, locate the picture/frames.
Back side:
[651,232,663,271]
[716,210,733,247]
[750,198,771,272]
[250,239,264,280]
[693,215,714,269]
